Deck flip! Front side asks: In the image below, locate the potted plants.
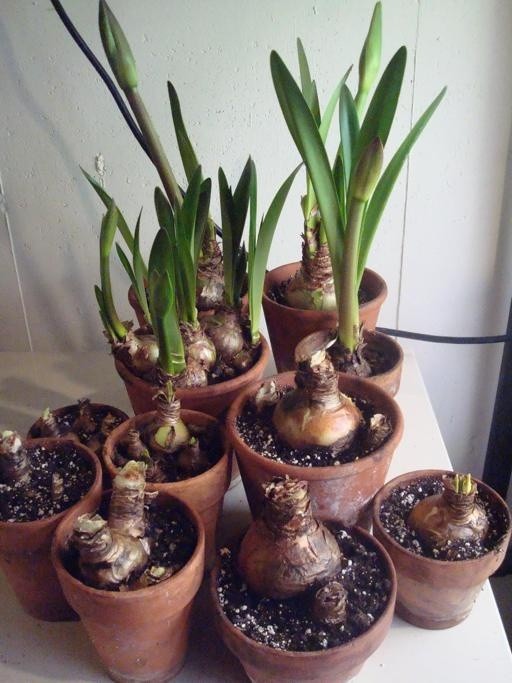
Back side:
[225,350,404,534]
[28,398,130,456]
[103,375,233,565]
[50,456,208,681]
[94,159,306,489]
[374,469,511,630]
[2,429,103,624]
[212,476,396,681]
[99,5,247,324]
[259,3,389,373]
[270,45,446,397]
